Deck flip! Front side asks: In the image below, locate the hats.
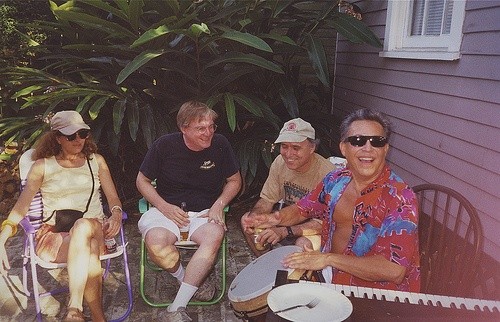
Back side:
[49,111,90,136]
[274,117,315,145]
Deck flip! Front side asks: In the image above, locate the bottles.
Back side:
[102,218,117,254]
[179,201,189,240]
[255,223,269,252]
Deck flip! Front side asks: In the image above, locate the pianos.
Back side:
[264,268,500,322]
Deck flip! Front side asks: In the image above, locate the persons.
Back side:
[136,101,243,322]
[241,117,336,281]
[241,108,422,294]
[0,111,123,322]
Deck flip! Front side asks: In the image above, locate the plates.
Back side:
[266,283,353,322]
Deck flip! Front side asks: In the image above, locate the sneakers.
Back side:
[162,306,194,322]
[178,264,216,302]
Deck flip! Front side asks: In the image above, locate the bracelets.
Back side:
[286,226,293,236]
[0,220,17,237]
[111,206,122,213]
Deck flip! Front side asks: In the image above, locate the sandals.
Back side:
[61,306,89,322]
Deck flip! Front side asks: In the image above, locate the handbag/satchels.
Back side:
[54,207,85,233]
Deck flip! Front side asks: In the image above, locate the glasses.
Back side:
[61,130,88,142]
[187,124,218,134]
[343,136,389,148]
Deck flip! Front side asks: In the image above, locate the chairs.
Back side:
[18,149,132,321]
[139,178,230,307]
[409,183,485,293]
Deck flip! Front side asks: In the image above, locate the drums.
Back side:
[227,245,321,322]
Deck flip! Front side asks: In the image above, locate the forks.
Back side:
[271,297,322,318]
